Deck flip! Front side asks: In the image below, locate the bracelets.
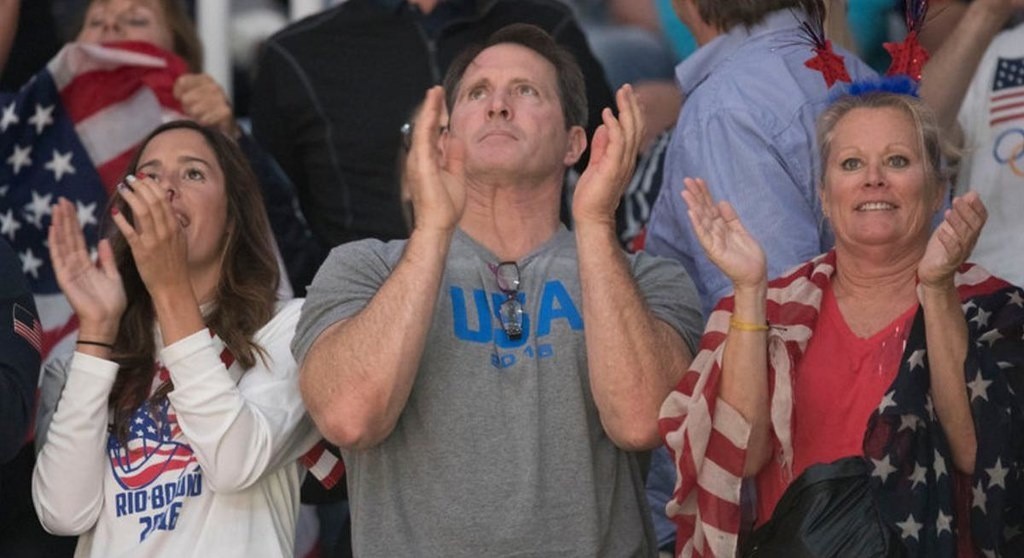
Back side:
[730,315,770,332]
[76,340,114,349]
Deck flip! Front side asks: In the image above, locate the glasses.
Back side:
[487,261,522,341]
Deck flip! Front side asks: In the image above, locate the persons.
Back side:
[0,0,1024,558]
[291,24,703,558]
[32,122,324,558]
[660,75,1023,558]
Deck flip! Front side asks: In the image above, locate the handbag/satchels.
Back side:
[738,456,905,558]
